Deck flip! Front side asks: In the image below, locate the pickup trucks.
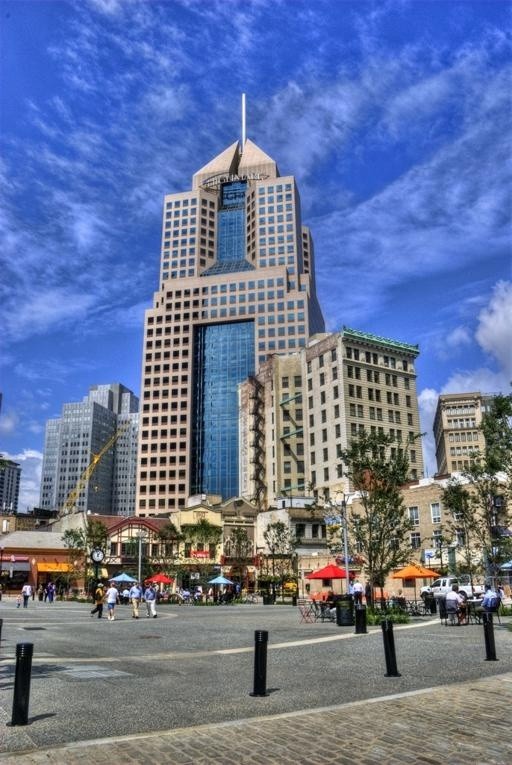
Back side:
[419,577,485,605]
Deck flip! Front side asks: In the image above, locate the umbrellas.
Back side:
[108,574,138,586]
[308,564,356,589]
[500,560,512,572]
[391,563,441,603]
[145,573,172,584]
[207,575,233,585]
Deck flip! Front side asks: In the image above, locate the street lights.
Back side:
[136,531,147,586]
[341,491,361,594]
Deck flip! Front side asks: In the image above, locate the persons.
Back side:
[347,578,507,625]
[325,590,338,619]
[16,582,240,622]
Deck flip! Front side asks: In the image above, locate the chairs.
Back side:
[295,593,496,628]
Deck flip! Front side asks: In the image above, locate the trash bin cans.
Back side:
[263,594,269,605]
[336,599,355,626]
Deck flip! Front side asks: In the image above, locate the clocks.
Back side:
[90,549,105,578]
